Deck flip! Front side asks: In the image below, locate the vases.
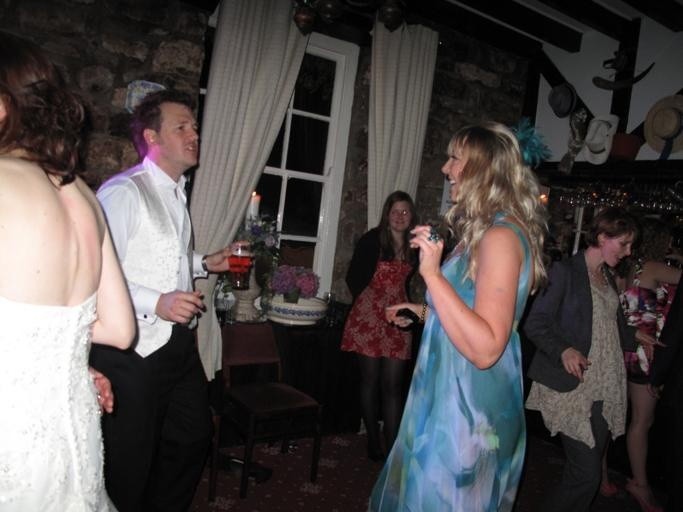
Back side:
[284,287,300,303]
[231,266,262,322]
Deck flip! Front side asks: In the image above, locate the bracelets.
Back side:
[201,255,207,272]
[419,301,426,322]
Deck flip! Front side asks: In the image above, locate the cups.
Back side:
[216,302,226,326]
[225,240,252,291]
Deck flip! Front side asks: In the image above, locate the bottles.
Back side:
[665,247,675,266]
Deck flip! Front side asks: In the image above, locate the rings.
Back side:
[426,231,439,240]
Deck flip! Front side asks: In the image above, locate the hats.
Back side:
[643,95,683,153]
[548,83,577,118]
[586,114,620,165]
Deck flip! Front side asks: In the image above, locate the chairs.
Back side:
[217,319,323,498]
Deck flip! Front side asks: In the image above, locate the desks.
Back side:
[220,319,328,446]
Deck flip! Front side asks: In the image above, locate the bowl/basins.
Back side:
[259,294,329,327]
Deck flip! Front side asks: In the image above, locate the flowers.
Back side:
[271,264,320,300]
[235,213,286,272]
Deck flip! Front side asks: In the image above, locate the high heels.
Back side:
[625,477,664,512]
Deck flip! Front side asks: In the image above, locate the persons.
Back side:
[97,88,255,512]
[339,191,422,463]
[0,29,135,512]
[87,365,114,417]
[522,206,666,511]
[598,218,682,511]
[647,251,682,511]
[367,120,552,511]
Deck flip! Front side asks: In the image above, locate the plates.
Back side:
[124,78,164,113]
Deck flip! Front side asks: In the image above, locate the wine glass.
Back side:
[552,183,680,216]
